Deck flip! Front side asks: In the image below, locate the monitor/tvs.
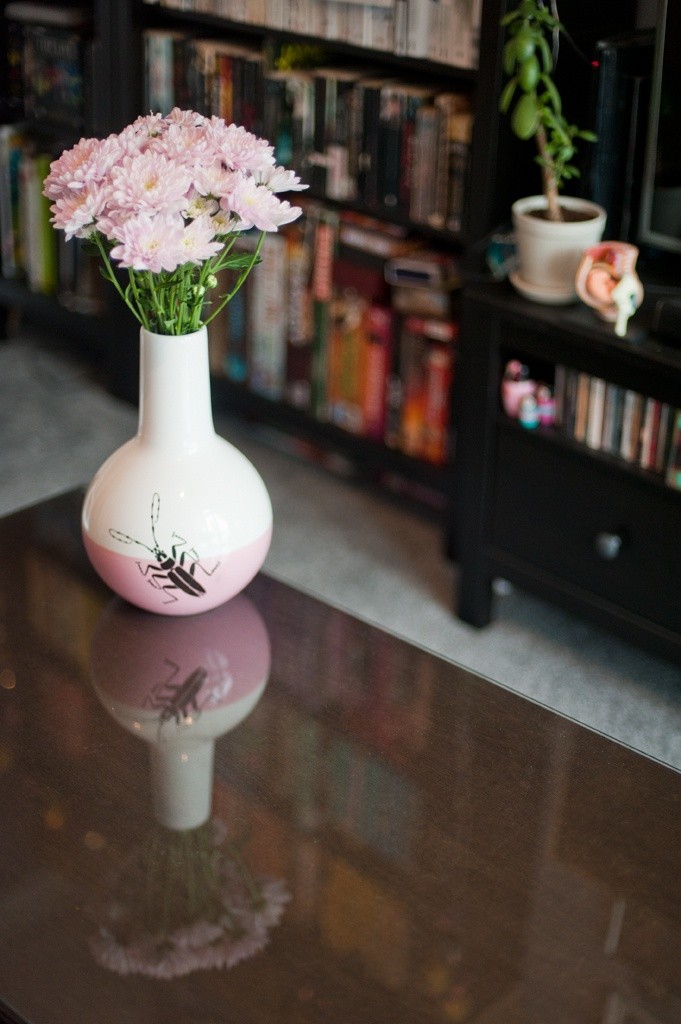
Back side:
[638,0,681,250]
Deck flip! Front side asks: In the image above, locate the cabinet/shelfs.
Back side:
[1,0,681,662]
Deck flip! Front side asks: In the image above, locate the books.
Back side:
[0,19,109,316]
[200,198,461,464]
[144,0,480,232]
[551,365,681,490]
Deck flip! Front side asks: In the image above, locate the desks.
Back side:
[0,483,681,1024]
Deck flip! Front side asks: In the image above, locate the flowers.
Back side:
[76,814,299,982]
[46,106,312,334]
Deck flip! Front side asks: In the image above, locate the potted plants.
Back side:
[487,0,608,307]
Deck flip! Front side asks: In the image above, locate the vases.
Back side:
[83,325,274,613]
[88,589,272,831]
